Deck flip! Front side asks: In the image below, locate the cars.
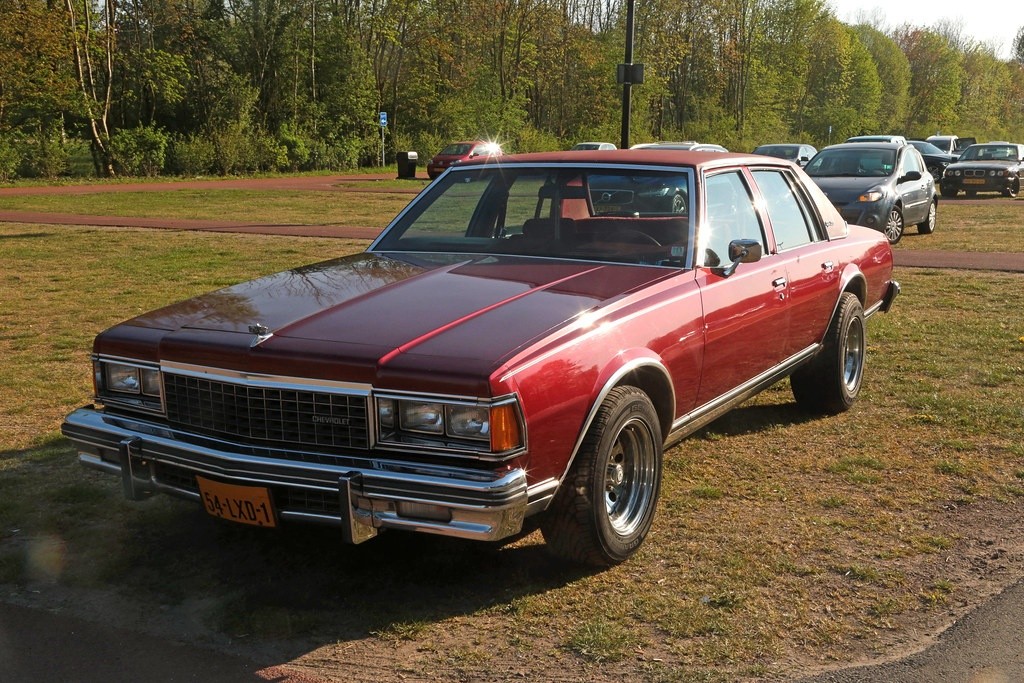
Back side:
[590,140,731,215]
[844,131,979,184]
[58,148,901,570]
[938,142,1024,199]
[427,140,503,182]
[799,142,939,245]
[571,140,617,151]
[751,144,823,170]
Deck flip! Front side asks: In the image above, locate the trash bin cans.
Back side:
[396,151,418,179]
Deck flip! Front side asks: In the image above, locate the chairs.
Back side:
[515,217,742,245]
[839,156,892,172]
[983,153,992,158]
